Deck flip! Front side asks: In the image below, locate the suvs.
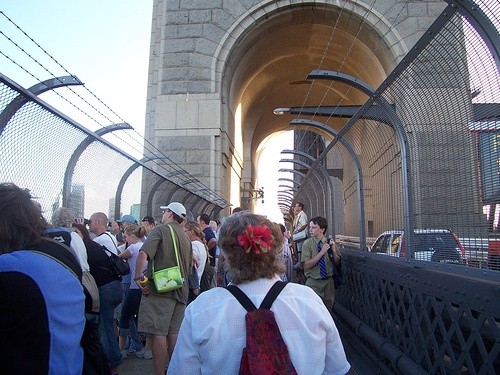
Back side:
[366,226,469,267]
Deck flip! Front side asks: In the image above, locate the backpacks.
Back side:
[92,246,129,280]
[216,280,298,374]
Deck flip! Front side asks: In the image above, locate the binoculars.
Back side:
[75,218,90,224]
[108,222,122,226]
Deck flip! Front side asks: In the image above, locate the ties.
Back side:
[317,241,327,280]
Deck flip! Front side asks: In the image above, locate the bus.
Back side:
[486,191,500,270]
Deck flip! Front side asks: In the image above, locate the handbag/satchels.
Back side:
[327,238,347,289]
[200,265,214,290]
[151,266,184,294]
[293,229,308,244]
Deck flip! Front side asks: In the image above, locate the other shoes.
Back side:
[126,347,144,355]
[121,350,126,358]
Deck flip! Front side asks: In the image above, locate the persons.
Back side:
[301,216,341,316]
[134,202,192,374]
[43,206,156,375]
[167,211,351,375]
[273,202,311,283]
[0,182,86,373]
[182,206,246,295]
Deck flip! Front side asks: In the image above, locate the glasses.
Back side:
[162,210,170,213]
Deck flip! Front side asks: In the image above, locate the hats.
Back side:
[116,215,135,223]
[160,202,186,220]
[142,216,154,223]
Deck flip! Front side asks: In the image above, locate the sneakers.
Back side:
[134,349,152,359]
